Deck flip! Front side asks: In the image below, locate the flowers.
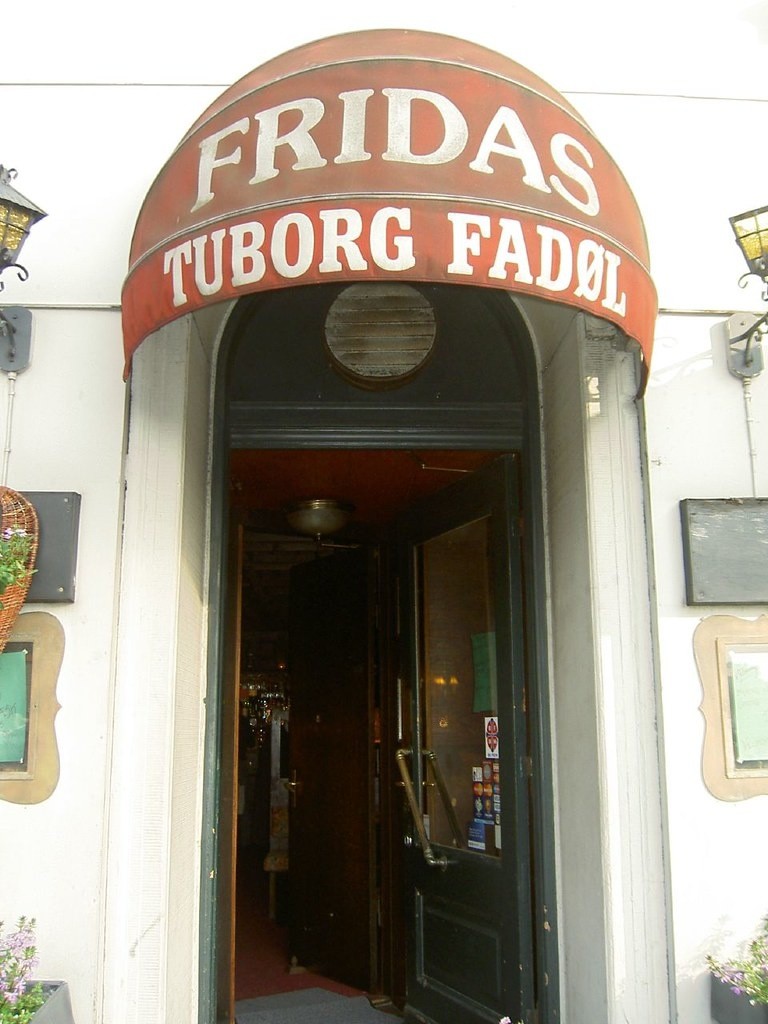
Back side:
[708,914,768,1005]
[0,915,43,1024]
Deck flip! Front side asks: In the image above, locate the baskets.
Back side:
[0,485,38,654]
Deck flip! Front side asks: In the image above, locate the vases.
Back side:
[26,980,75,1024]
[710,972,768,1024]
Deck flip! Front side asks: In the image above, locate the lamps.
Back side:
[0,163,48,372]
[285,498,400,577]
[723,204,768,380]
